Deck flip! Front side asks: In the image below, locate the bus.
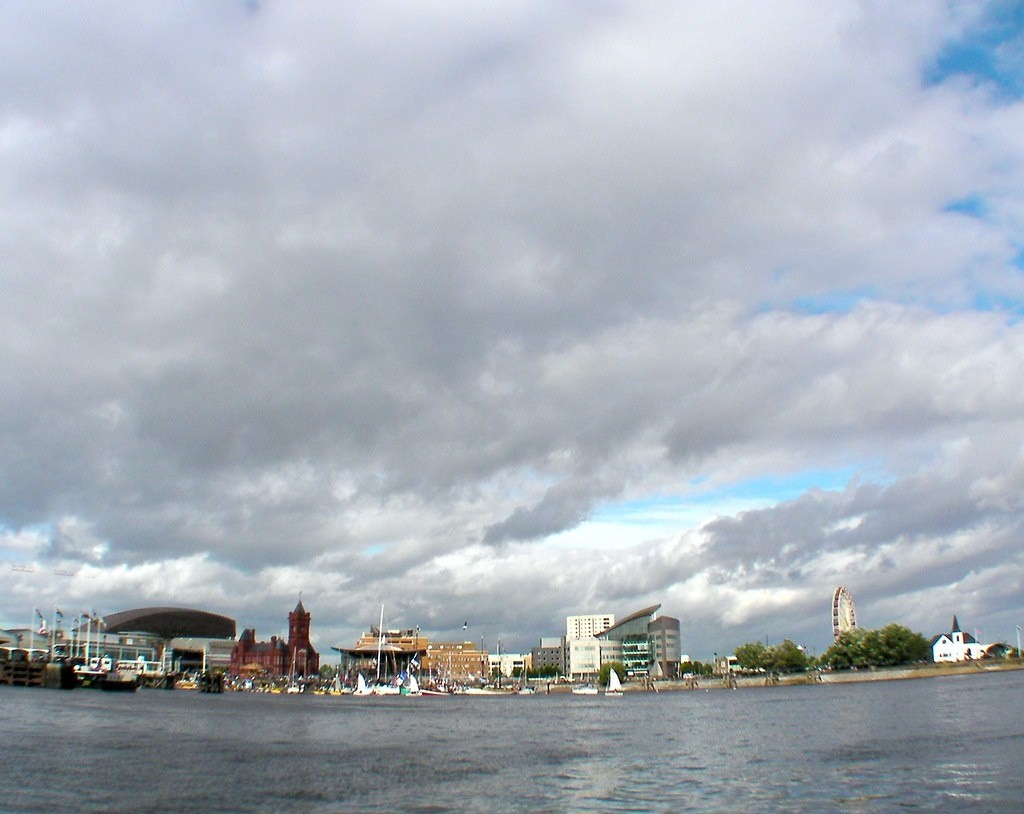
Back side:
[0,647,50,662]
[90,657,114,672]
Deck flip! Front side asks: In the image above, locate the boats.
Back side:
[572,685,598,695]
[97,678,139,692]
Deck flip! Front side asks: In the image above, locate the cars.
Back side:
[551,676,576,684]
[476,677,489,684]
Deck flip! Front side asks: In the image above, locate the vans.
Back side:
[683,674,694,680]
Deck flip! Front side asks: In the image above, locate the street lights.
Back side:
[1017,625,1022,656]
[713,652,717,678]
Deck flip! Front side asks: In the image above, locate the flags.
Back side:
[975,629,983,635]
[56,608,64,617]
[35,609,42,618]
[73,618,79,625]
[80,608,97,618]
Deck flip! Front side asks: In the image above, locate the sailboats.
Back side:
[605,665,624,696]
[174,604,539,695]
